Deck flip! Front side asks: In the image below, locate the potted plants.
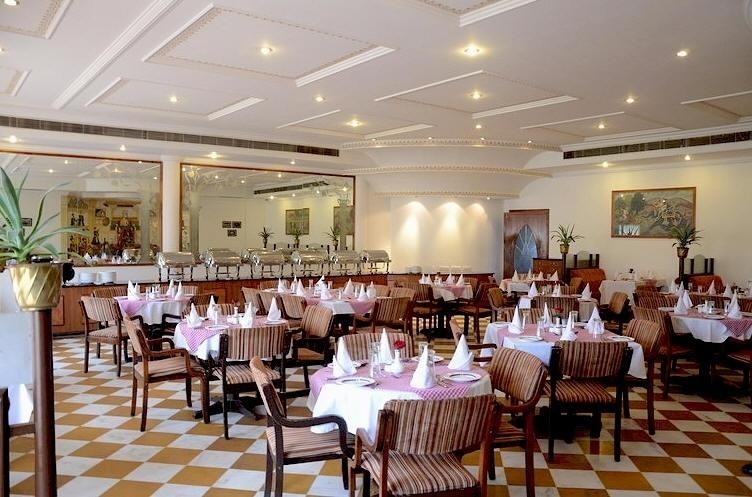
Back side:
[0,167,94,311]
[669,224,704,258]
[551,223,584,253]
[257,227,274,242]
[291,227,302,244]
[323,227,339,246]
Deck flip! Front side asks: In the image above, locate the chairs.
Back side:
[549,341,634,461]
[348,393,498,495]
[249,356,350,495]
[455,347,549,497]
[79,276,751,442]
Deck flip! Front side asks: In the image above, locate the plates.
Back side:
[182,307,285,330]
[79,269,117,285]
[114,284,194,302]
[493,322,511,328]
[607,335,635,342]
[338,376,375,388]
[444,373,482,382]
[514,270,564,297]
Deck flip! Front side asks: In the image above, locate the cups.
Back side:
[358,341,452,388]
[265,278,378,304]
[518,305,602,343]
[657,279,752,320]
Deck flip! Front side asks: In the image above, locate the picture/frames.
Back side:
[611,187,696,239]
[222,221,241,237]
[285,208,309,235]
[22,218,32,226]
[334,206,353,235]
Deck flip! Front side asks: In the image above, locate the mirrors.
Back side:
[179,163,356,264]
[0,150,163,267]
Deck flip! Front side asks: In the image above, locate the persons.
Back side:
[91,226,100,245]
[71,213,76,225]
[70,238,76,252]
[79,238,87,257]
